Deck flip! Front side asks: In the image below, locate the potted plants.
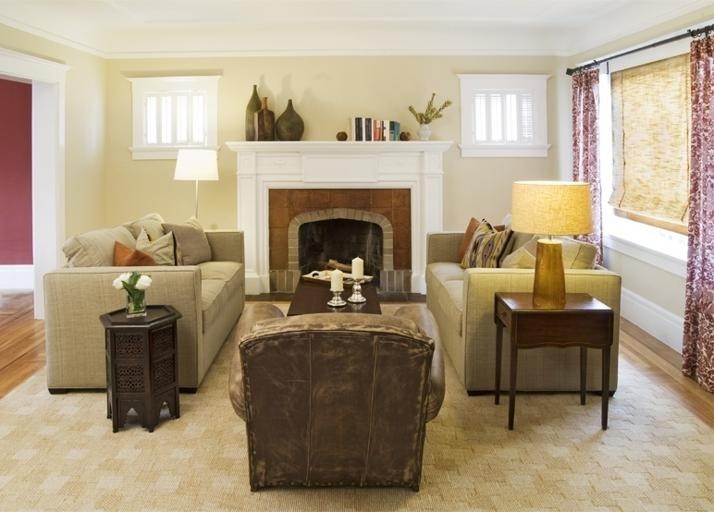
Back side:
[406,89,451,140]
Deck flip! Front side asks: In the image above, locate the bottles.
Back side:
[272,97,304,141]
[244,84,261,142]
[253,95,275,140]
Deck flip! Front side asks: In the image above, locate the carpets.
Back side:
[0,298,713,510]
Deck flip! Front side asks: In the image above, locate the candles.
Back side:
[330,268,345,290]
[351,256,365,279]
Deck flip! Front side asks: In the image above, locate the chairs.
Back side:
[228,299,446,492]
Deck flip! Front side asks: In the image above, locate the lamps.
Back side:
[170,147,221,220]
[511,181,593,311]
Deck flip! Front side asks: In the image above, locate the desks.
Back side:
[97,306,182,434]
[493,289,613,434]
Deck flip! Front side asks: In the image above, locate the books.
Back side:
[351,115,400,141]
[301,269,373,289]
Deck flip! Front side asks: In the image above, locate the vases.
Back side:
[126,293,146,318]
[244,84,303,141]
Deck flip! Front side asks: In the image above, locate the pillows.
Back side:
[134,223,176,266]
[500,246,536,269]
[162,217,214,265]
[136,226,149,249]
[457,219,513,271]
[111,238,158,266]
[457,215,504,257]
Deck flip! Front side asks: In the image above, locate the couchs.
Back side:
[42,209,248,393]
[422,213,619,398]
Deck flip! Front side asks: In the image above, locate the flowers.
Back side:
[112,271,151,310]
